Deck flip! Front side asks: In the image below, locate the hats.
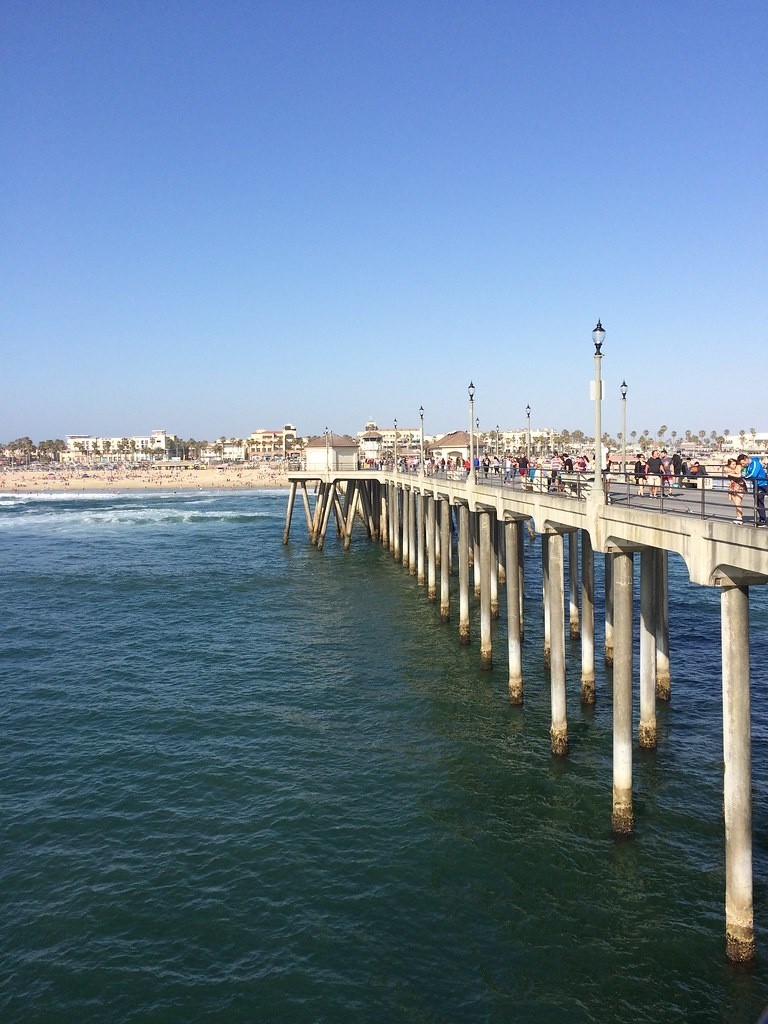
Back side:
[677,450,681,453]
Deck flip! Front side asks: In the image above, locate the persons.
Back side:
[503,452,614,494]
[364,457,445,477]
[447,453,500,479]
[635,450,708,499]
[723,454,768,528]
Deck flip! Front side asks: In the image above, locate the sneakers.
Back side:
[733,518,743,525]
[752,520,766,527]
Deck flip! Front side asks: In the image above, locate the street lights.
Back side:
[419,405,425,476]
[467,380,477,485]
[526,404,531,459]
[588,317,607,504]
[393,417,397,473]
[617,378,629,481]
[475,417,480,459]
[496,424,499,457]
[325,426,329,470]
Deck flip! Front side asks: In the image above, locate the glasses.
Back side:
[640,457,644,458]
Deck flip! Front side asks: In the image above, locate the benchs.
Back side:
[682,477,713,490]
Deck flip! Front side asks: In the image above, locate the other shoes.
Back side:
[637,491,666,499]
[668,493,673,498]
